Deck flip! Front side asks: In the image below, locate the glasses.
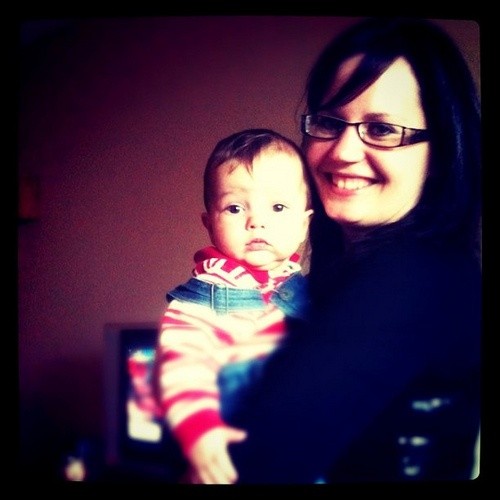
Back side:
[299,113,430,147]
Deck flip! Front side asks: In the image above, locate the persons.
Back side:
[134,17,481,484]
[154,128,315,484]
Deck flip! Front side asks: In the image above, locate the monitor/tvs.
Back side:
[104,322,170,468]
